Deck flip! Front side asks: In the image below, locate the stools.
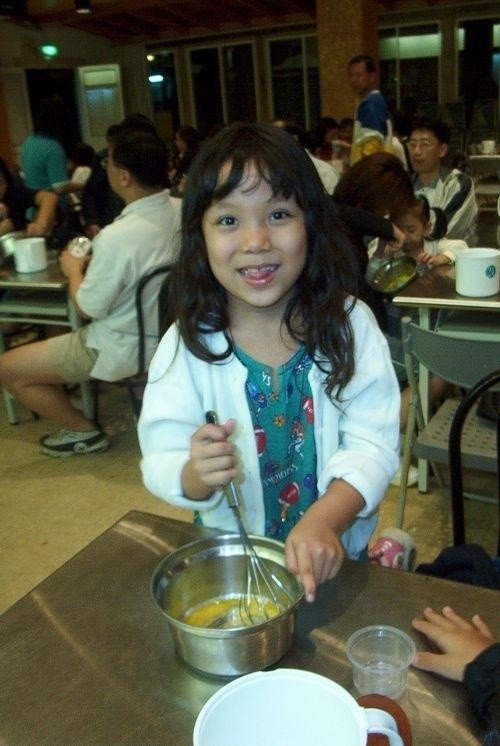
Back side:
[476,184,500,220]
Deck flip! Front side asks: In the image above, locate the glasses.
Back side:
[406,139,438,151]
[100,158,120,169]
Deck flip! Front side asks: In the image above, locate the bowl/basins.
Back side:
[369,254,420,299]
[148,532,307,677]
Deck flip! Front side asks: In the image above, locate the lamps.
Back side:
[146,71,166,87]
[41,44,59,57]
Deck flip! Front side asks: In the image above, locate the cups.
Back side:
[454,246,499,299]
[345,623,418,701]
[13,237,49,274]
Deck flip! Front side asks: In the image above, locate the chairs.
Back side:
[411,369,498,592]
[91,263,178,429]
[395,317,500,530]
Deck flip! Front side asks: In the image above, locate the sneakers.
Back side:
[369,524,420,572]
[389,457,418,486]
[38,427,112,457]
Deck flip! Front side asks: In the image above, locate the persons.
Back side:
[409,605,500,746]
[19,124,86,206]
[368,195,469,267]
[407,118,478,253]
[169,125,198,182]
[0,130,185,458]
[0,160,58,237]
[347,54,393,165]
[334,152,413,254]
[272,116,354,195]
[137,122,402,604]
